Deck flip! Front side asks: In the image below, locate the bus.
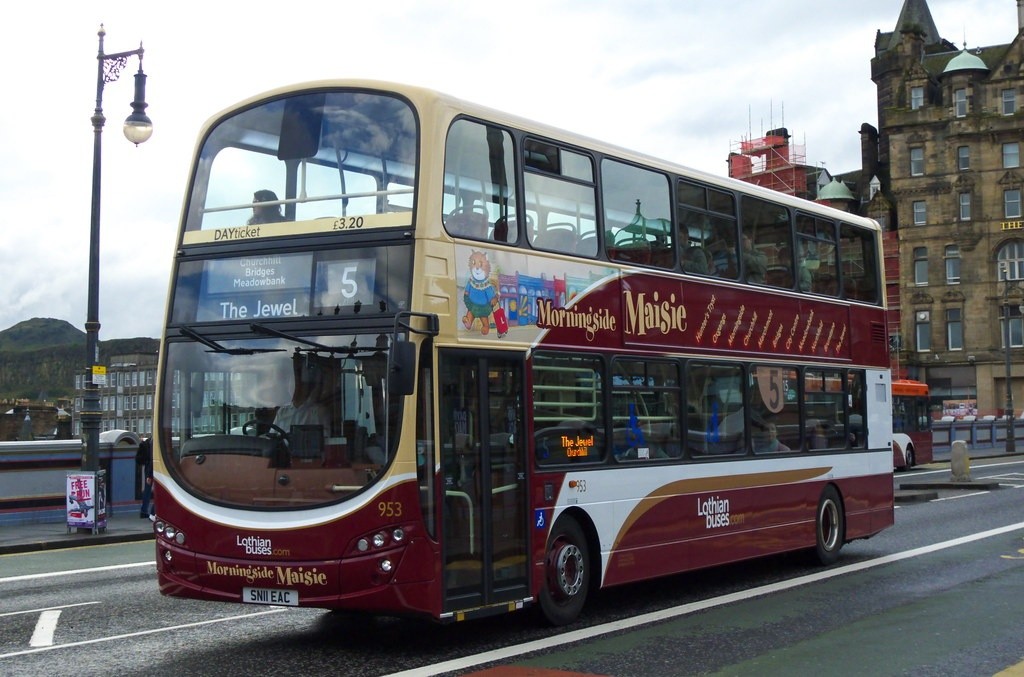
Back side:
[146,77,894,621]
[755,368,935,471]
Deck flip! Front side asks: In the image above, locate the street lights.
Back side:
[75,20,154,532]
[1001,268,1024,453]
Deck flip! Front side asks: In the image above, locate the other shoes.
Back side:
[149,514,155,521]
[140,513,150,518]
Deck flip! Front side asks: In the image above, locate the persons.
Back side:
[622,421,670,460]
[778,247,812,293]
[359,351,387,439]
[268,366,333,446]
[245,190,289,226]
[678,221,711,275]
[753,419,791,454]
[727,229,769,285]
[805,417,829,450]
[489,366,520,541]
[135,434,154,519]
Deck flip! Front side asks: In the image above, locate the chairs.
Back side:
[444,203,857,300]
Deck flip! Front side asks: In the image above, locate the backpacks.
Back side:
[135,437,151,464]
